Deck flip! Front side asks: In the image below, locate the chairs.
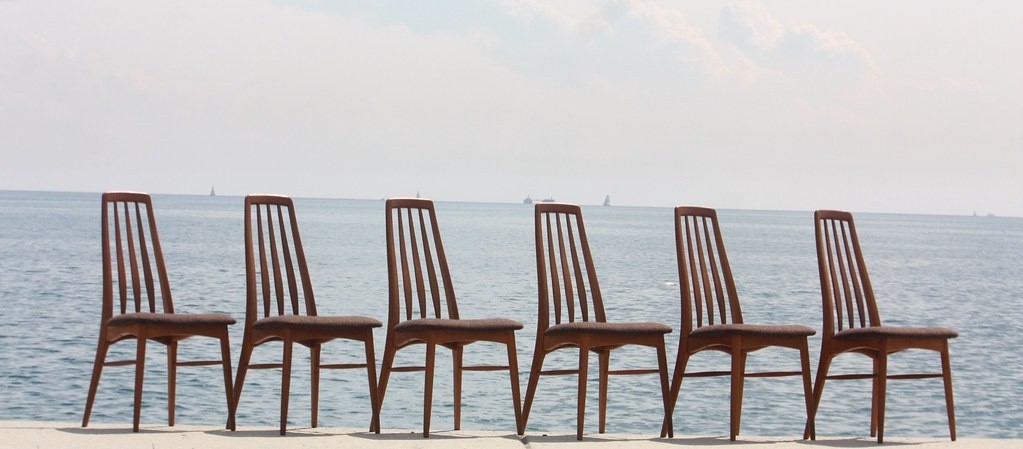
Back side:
[81,192,237,432]
[520,203,674,439]
[803,210,958,445]
[225,194,380,434]
[369,198,520,433]
[661,206,816,442]
